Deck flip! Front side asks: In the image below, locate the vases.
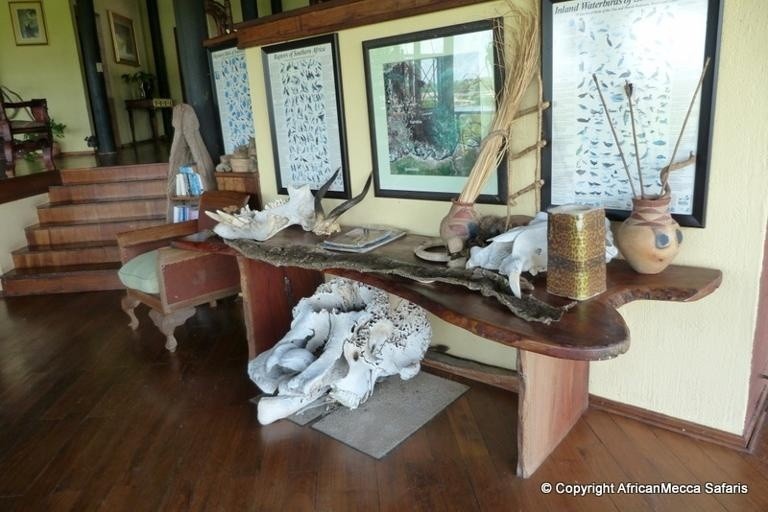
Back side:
[615,193,683,275]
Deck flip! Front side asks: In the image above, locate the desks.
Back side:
[123,98,174,154]
[164,221,724,482]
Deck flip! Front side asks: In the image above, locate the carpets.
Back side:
[246,369,472,462]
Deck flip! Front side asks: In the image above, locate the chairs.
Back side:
[0,84,56,181]
[112,190,251,354]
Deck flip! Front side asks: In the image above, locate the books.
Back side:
[319,223,409,255]
[171,161,205,226]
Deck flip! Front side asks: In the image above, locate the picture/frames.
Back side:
[8,1,48,47]
[258,31,352,200]
[106,8,141,68]
[201,37,256,164]
[360,14,510,207]
[537,0,726,229]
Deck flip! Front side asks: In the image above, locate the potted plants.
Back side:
[228,142,255,174]
[22,119,66,161]
